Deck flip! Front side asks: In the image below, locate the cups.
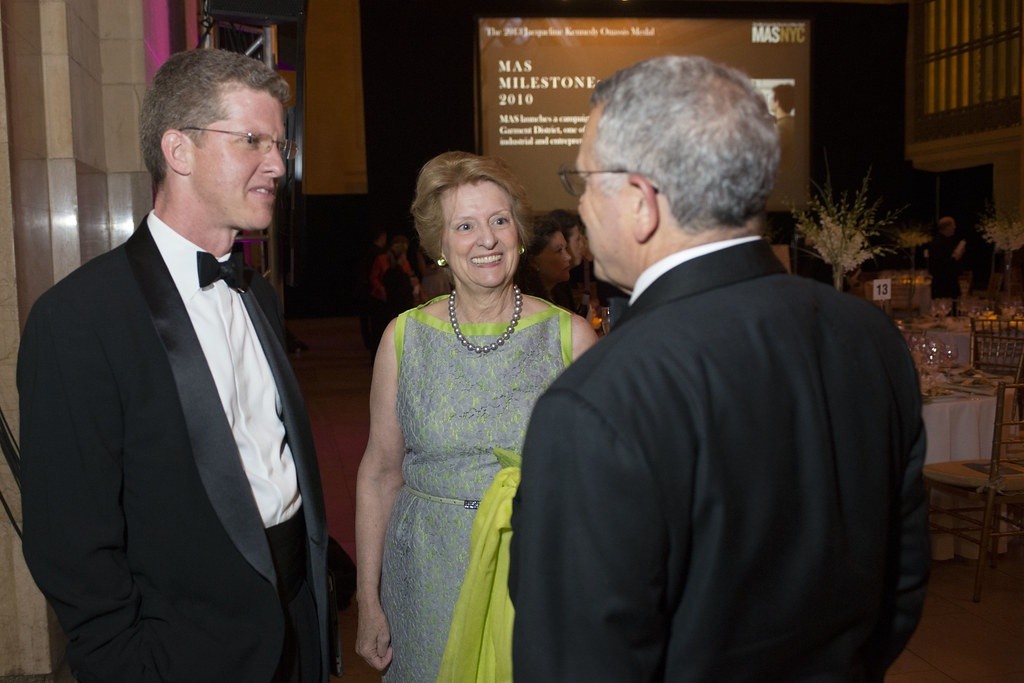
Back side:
[602,307,609,335]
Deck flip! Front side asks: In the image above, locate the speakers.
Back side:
[207,0,308,25]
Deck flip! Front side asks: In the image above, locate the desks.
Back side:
[918,363,1020,561]
[894,316,1024,374]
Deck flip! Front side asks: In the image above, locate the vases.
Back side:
[1004,252,1012,296]
[830,272,846,292]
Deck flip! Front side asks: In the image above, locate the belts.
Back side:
[404,484,482,509]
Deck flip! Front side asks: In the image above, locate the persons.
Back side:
[360,225,451,337]
[16,51,339,683]
[355,154,597,683]
[512,209,594,319]
[928,216,966,317]
[508,55,931,683]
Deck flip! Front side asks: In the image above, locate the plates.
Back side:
[922,390,955,398]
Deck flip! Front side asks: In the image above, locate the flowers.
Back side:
[978,203,1024,251]
[779,154,898,274]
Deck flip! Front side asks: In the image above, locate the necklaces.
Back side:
[448,284,523,353]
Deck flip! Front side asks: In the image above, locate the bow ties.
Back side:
[198,250,245,288]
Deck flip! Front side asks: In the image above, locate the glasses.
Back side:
[178,126,298,160]
[559,162,658,196]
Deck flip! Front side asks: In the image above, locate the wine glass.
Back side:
[929,297,953,324]
[908,333,958,384]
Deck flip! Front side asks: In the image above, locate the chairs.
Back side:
[969,318,1024,383]
[922,382,1024,605]
